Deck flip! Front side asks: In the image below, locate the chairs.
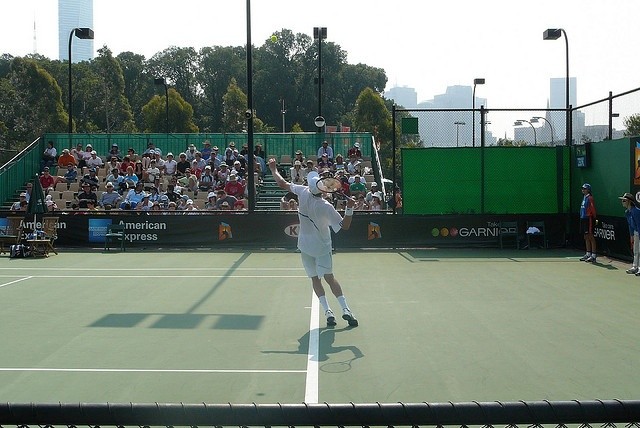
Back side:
[103,223,128,252]
[497,221,522,249]
[1,216,23,256]
[9,153,255,210]
[269,154,376,211]
[29,216,59,258]
[526,220,546,252]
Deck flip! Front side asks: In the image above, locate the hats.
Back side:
[306,159,313,163]
[111,156,118,160]
[294,161,301,165]
[371,181,377,187]
[91,150,97,154]
[202,140,212,145]
[307,171,324,194]
[227,174,239,182]
[229,141,236,146]
[179,194,189,200]
[112,143,118,146]
[62,148,69,153]
[19,192,26,196]
[86,143,92,147]
[234,200,244,206]
[618,192,637,206]
[179,152,187,159]
[348,153,356,157]
[219,161,229,167]
[243,144,248,147]
[207,192,218,201]
[230,170,239,174]
[166,152,174,157]
[295,149,303,154]
[89,168,96,172]
[372,192,381,200]
[581,183,592,190]
[321,140,328,144]
[205,165,212,169]
[354,142,360,147]
[105,181,114,188]
[161,195,168,200]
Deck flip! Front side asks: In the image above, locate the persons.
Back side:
[280,193,291,210]
[134,193,154,209]
[149,149,155,159]
[268,158,358,327]
[10,192,26,210]
[348,168,367,187]
[181,175,199,194]
[199,166,216,192]
[148,184,161,203]
[98,182,123,208]
[134,159,143,180]
[216,191,238,209]
[200,140,212,160]
[618,193,640,276]
[106,143,123,162]
[121,182,146,208]
[240,143,248,156]
[393,186,402,214]
[148,151,165,170]
[142,143,162,170]
[26,183,32,203]
[47,187,54,194]
[347,154,358,174]
[40,141,57,168]
[123,148,140,161]
[334,170,348,183]
[185,199,198,210]
[166,176,181,194]
[146,161,160,187]
[302,160,317,185]
[205,192,217,210]
[212,146,223,162]
[84,144,92,159]
[177,168,198,186]
[224,148,237,161]
[366,182,383,205]
[354,161,374,175]
[231,160,246,182]
[233,149,247,160]
[317,141,333,164]
[124,166,139,189]
[206,160,217,179]
[349,177,367,199]
[369,192,383,210]
[47,202,60,211]
[289,198,297,210]
[76,181,97,208]
[293,150,307,167]
[177,152,190,176]
[231,169,243,182]
[159,152,177,175]
[334,176,349,208]
[86,199,95,208]
[163,185,183,201]
[177,195,189,209]
[347,142,361,158]
[106,169,125,190]
[333,154,347,170]
[217,161,230,183]
[54,162,77,185]
[225,174,244,197]
[234,200,247,210]
[106,156,121,176]
[180,187,189,195]
[58,149,77,168]
[325,192,334,205]
[79,168,100,191]
[235,193,245,200]
[85,150,102,168]
[168,201,177,210]
[121,157,134,175]
[229,141,236,150]
[579,183,596,262]
[319,168,333,178]
[290,161,305,184]
[16,201,27,210]
[318,153,333,178]
[254,143,264,159]
[206,152,221,165]
[253,158,263,177]
[185,143,202,166]
[153,204,160,210]
[71,143,86,168]
[190,159,202,179]
[39,166,54,194]
[353,194,369,210]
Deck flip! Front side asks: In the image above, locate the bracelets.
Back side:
[345,206,353,216]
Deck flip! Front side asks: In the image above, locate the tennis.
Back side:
[271,36,278,43]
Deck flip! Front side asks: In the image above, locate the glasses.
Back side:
[107,185,113,187]
[44,169,50,171]
[68,165,72,167]
[322,155,326,157]
[27,186,33,188]
[222,205,228,207]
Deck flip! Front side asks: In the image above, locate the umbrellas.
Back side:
[25,173,48,248]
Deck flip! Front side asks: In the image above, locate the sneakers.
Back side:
[580,254,589,260]
[326,308,337,327]
[585,255,596,262]
[342,307,358,326]
[636,270,640,276]
[626,267,638,273]
